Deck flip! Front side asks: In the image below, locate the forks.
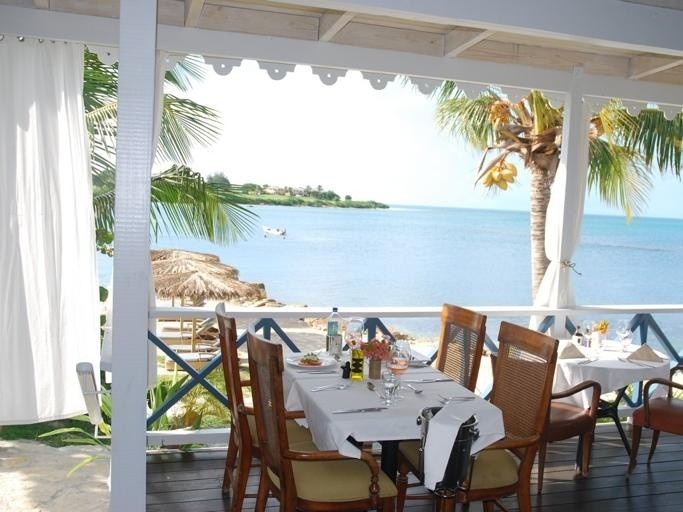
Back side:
[408,384,423,393]
[309,380,355,391]
[436,393,475,404]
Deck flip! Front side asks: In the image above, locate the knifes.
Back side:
[296,369,337,374]
[578,357,598,365]
[332,408,389,414]
[403,378,453,382]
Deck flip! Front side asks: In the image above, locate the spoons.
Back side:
[368,382,383,398]
[619,355,657,368]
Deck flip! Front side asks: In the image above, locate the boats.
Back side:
[262,225,287,239]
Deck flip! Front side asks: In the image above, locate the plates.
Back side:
[286,354,337,367]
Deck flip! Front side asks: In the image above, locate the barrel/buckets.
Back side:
[416,406,479,491]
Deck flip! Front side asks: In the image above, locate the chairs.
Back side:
[378,301,487,486]
[487,348,602,479]
[213,300,318,510]
[624,363,683,477]
[241,322,396,510]
[395,320,559,511]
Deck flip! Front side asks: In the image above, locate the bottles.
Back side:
[327,307,364,380]
[573,325,591,347]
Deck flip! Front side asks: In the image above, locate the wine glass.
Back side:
[384,341,412,407]
[615,319,633,353]
